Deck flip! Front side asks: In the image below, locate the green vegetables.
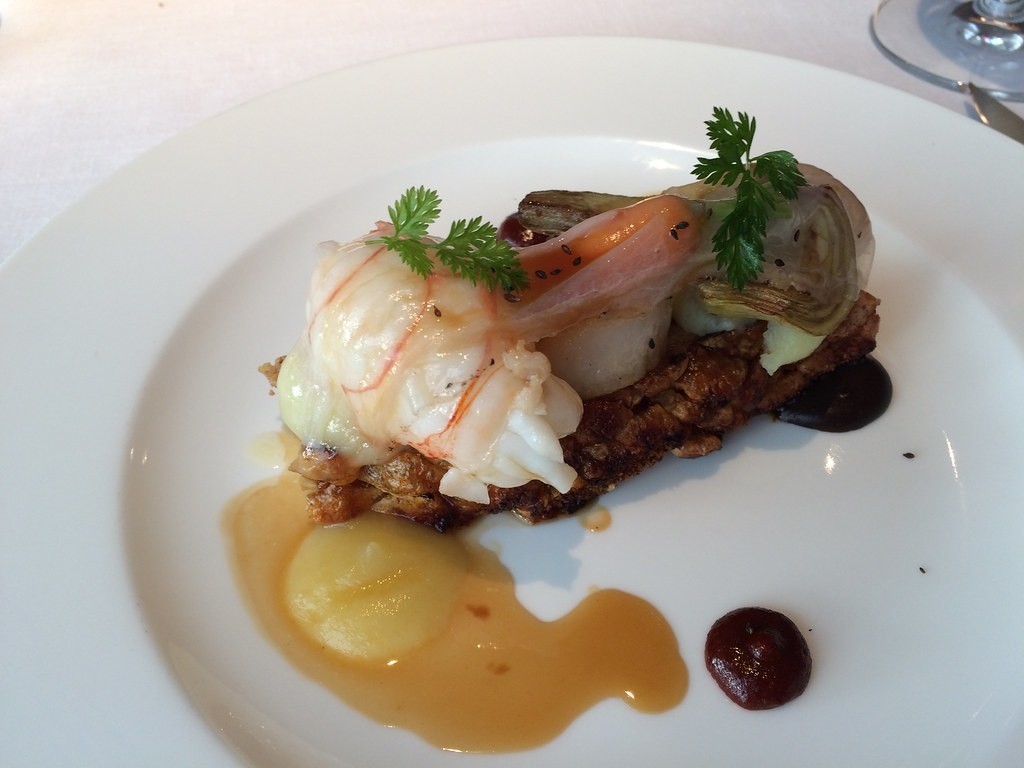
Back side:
[361,185,532,296]
[690,107,807,293]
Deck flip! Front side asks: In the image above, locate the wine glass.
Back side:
[873,0,1024,100]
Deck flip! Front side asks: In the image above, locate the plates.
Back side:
[1,37,1024,768]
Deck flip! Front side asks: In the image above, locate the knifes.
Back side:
[967,82,1024,146]
